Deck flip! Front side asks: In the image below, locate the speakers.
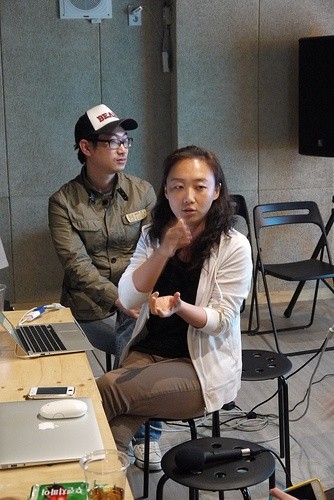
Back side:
[298,35,334,157]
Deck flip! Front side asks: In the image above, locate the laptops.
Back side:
[0,398,105,469]
[0,311,93,358]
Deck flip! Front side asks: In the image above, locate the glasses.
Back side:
[97,137,133,150]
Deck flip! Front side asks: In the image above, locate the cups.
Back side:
[0,284,8,313]
[80,448,130,500]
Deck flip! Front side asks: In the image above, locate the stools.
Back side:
[155,437,276,500]
[214,349,292,488]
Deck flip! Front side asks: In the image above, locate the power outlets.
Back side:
[128,5,142,26]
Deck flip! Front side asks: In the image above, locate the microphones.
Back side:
[175,445,265,474]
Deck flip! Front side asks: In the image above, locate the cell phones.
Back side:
[284,478,326,500]
[28,386,75,399]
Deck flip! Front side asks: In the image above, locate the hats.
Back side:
[74,104,138,150]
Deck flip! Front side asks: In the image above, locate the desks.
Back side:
[0,307,134,500]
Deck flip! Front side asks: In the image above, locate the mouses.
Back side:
[40,399,87,419]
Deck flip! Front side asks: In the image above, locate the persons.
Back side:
[96,145,253,490]
[49,104,162,472]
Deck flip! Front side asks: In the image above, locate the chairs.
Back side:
[248,201,334,358]
[227,195,260,335]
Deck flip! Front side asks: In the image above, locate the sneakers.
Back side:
[127,441,135,465]
[134,441,162,470]
